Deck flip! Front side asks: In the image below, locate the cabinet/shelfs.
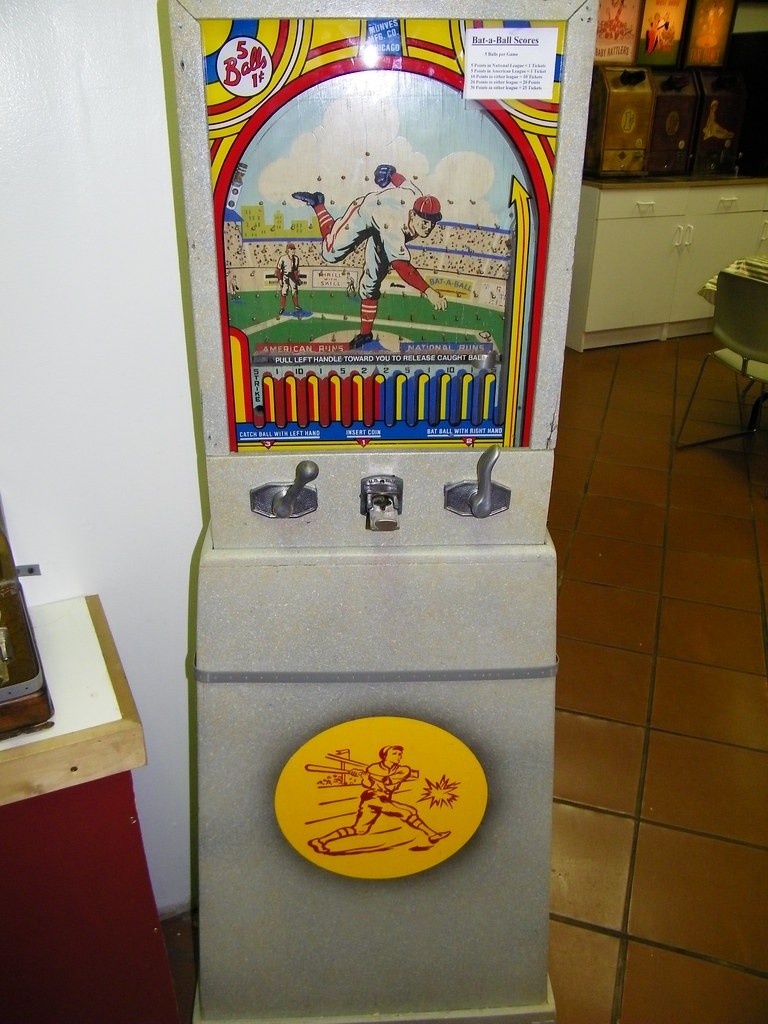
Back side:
[565,185,768,353]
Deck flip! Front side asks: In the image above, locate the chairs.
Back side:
[674,271,768,451]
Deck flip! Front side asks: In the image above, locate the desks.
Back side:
[0,593,183,1024]
